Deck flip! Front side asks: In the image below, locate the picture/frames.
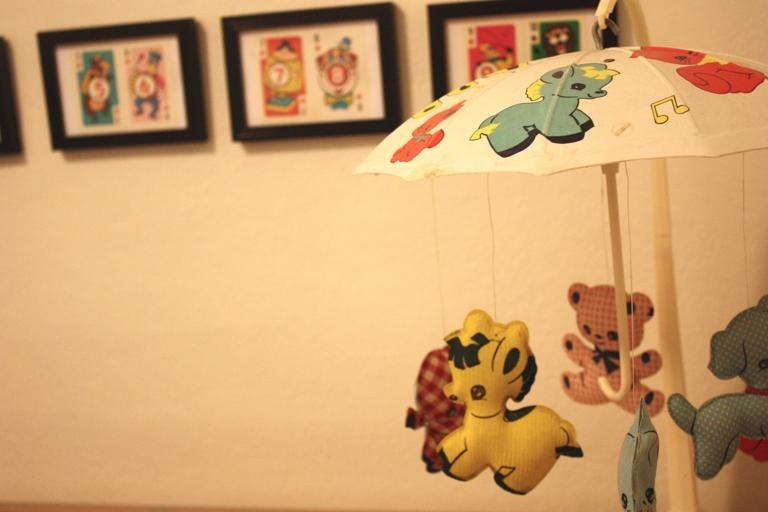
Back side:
[0,36,22,155]
[427,0,621,102]
[221,3,402,142]
[36,18,208,150]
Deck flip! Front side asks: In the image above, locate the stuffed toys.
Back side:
[664,292,767,480]
[433,306,585,496]
[407,342,464,476]
[561,280,662,416]
[616,398,658,512]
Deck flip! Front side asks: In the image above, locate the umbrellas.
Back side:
[351,46,768,405]
